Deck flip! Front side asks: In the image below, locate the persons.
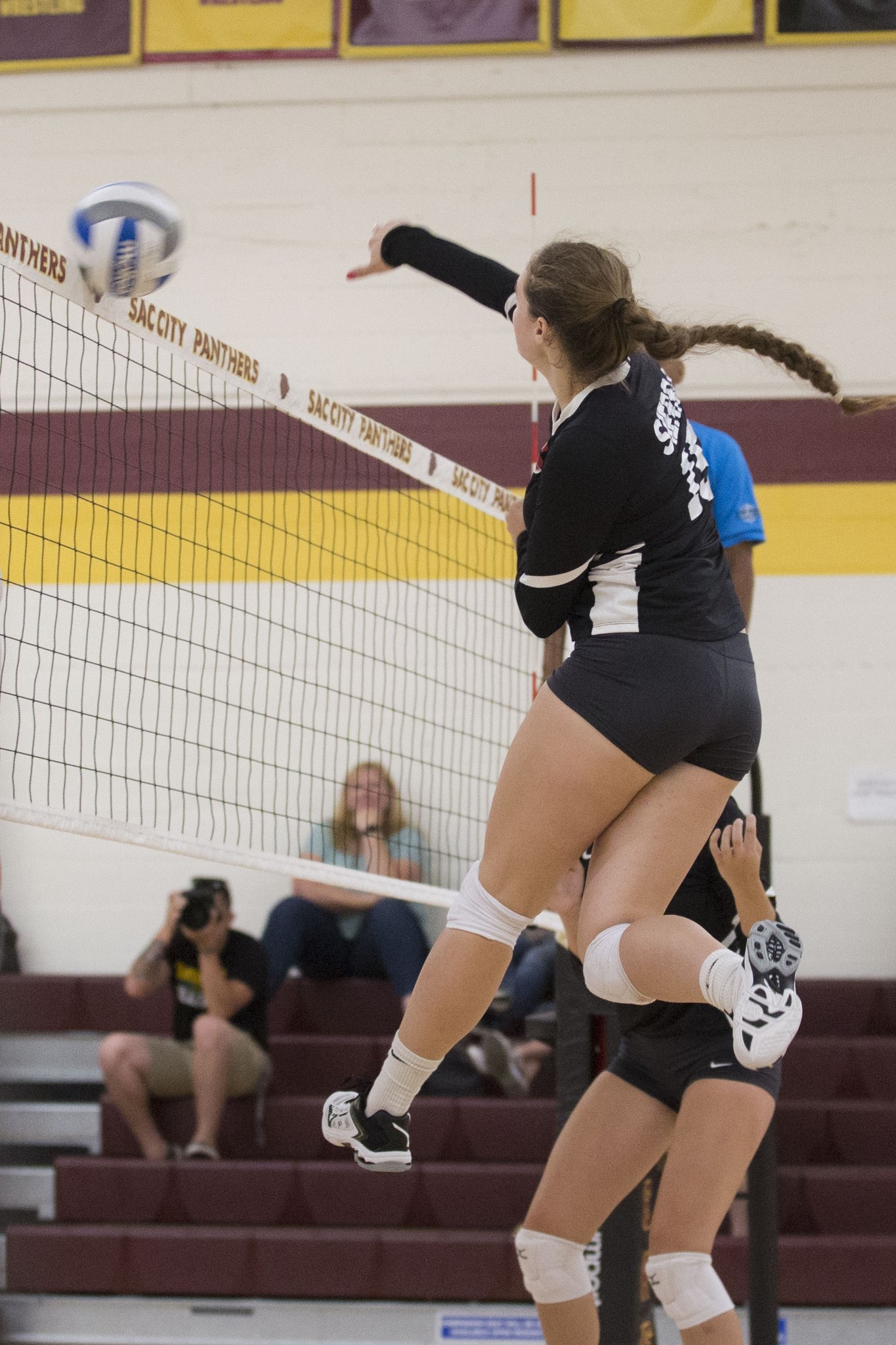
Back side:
[98,875,270,1161]
[321,219,895,1174]
[258,760,596,1095]
[513,788,782,1345]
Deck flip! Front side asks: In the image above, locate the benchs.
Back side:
[0,967,896,1345]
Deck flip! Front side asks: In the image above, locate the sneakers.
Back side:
[731,919,804,1071]
[321,1090,413,1172]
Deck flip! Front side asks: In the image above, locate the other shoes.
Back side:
[468,1030,530,1101]
[153,1141,220,1160]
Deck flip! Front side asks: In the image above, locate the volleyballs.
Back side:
[69,182,186,297]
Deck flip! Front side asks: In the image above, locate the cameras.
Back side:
[179,890,216,930]
